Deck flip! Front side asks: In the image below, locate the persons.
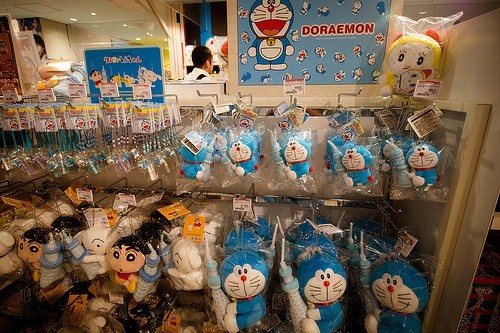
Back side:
[181,46,217,81]
[23,18,48,60]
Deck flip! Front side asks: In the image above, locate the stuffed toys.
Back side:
[176,129,443,193]
[1,211,430,333]
[375,30,446,103]
